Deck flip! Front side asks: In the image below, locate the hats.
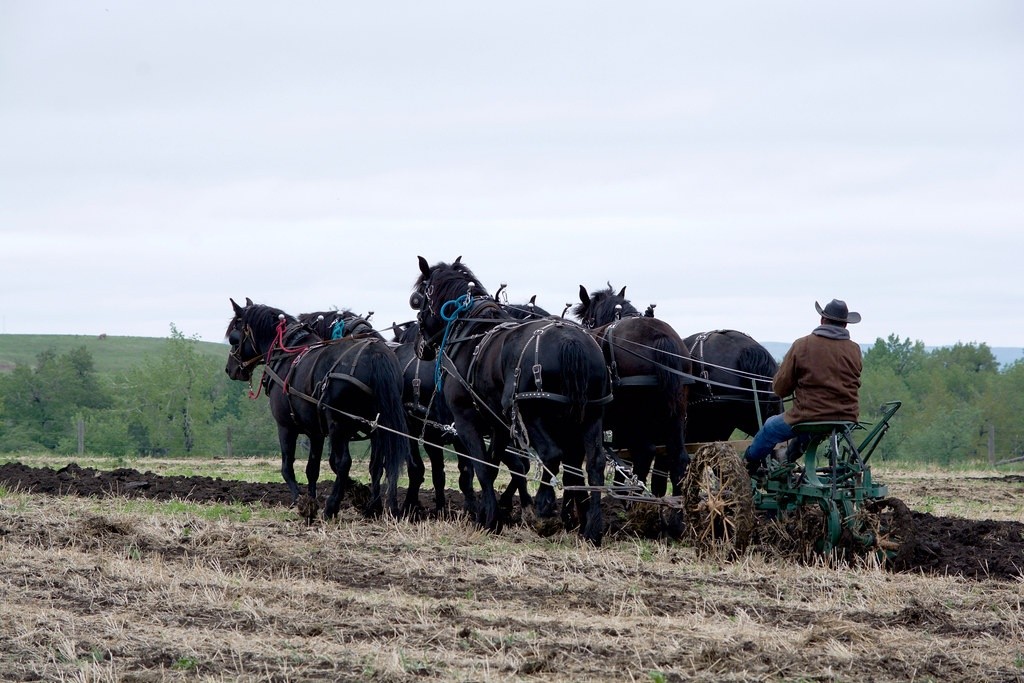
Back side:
[815,299,861,323]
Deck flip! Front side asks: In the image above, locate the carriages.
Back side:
[224,252,918,579]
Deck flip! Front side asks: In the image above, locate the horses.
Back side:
[223,256,786,532]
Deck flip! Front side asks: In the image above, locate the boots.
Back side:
[742,445,762,479]
[771,437,806,465]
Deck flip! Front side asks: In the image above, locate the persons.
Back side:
[741,298,863,479]
